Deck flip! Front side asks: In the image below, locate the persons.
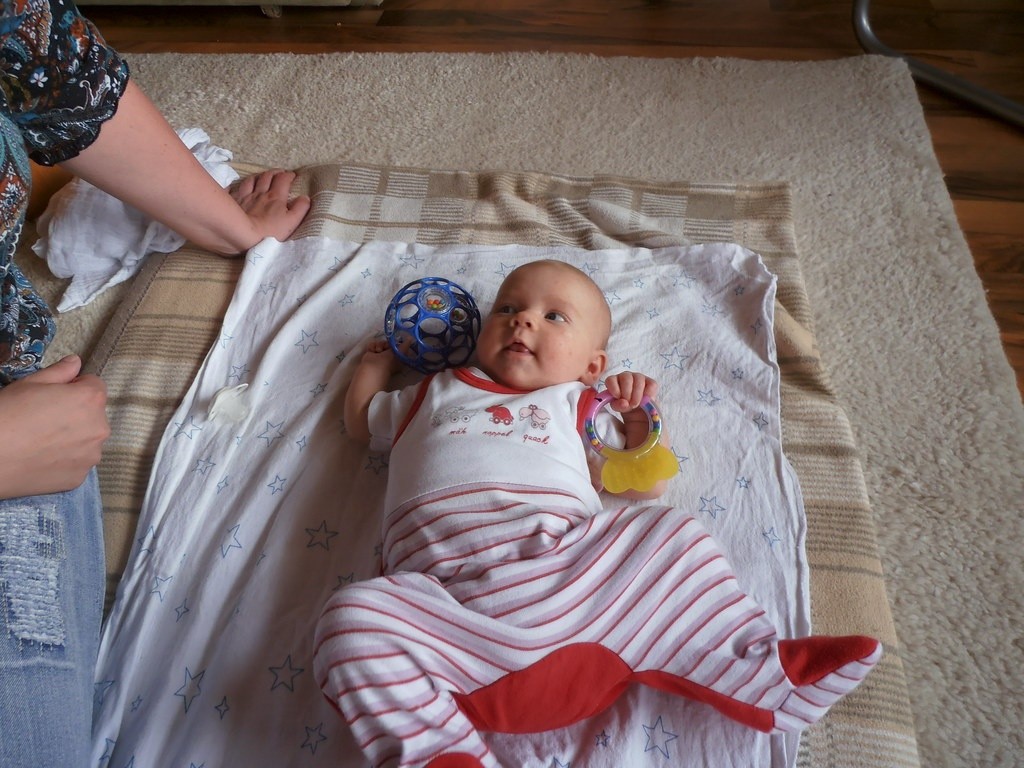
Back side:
[312,259,882,767]
[0,1,311,768]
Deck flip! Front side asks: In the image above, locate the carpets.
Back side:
[0,53,1024,768]
